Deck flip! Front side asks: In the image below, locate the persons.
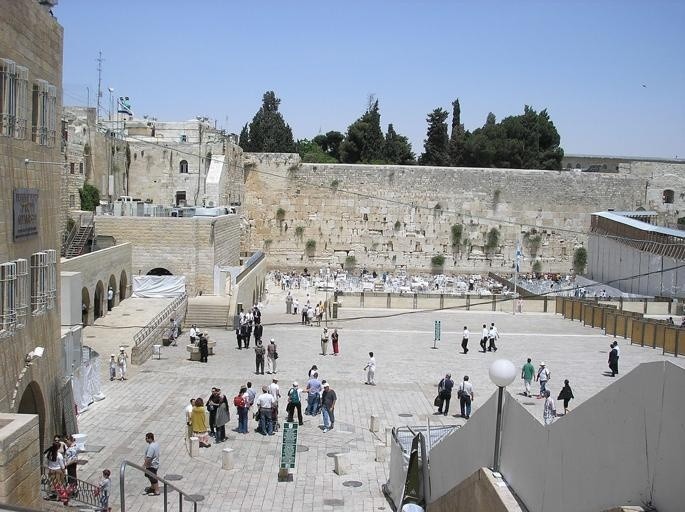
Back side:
[109,347,128,380]
[364,351,376,384]
[321,327,339,356]
[42,434,77,503]
[274,270,324,325]
[186,379,280,446]
[170,318,208,362]
[669,315,674,325]
[521,358,574,425]
[98,469,111,511]
[461,323,499,353]
[682,316,685,325]
[288,365,338,432]
[523,272,605,297]
[607,344,617,377]
[143,432,160,495]
[108,287,113,311]
[613,341,620,374]
[235,306,277,375]
[438,373,473,418]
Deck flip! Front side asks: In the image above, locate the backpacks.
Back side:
[289,387,301,402]
[234,397,246,407]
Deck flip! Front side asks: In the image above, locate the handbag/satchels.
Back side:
[439,386,446,392]
[118,360,125,367]
[434,397,441,406]
[457,390,471,401]
[256,354,263,360]
[274,352,278,359]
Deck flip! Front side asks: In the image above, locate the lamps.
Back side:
[25,345,44,363]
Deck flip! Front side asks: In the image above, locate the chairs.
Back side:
[152,345,161,360]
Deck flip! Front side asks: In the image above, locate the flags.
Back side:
[516,240,522,272]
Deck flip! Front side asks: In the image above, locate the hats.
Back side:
[271,339,274,343]
[322,327,328,332]
[119,348,124,353]
[240,365,331,391]
[110,353,115,357]
[539,362,545,367]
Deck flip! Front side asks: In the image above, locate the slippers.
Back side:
[148,490,159,494]
[145,486,152,492]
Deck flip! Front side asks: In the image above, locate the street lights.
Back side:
[488,357,517,472]
[108,87,113,121]
